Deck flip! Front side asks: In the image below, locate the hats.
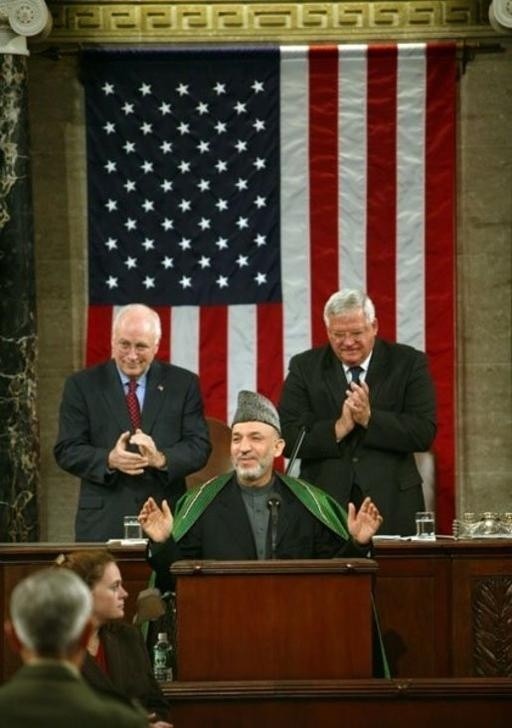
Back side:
[232,391,281,436]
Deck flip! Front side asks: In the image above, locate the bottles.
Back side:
[153,632,177,685]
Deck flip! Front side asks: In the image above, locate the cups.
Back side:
[413,511,437,540]
[123,515,143,539]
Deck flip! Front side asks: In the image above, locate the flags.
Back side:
[74,40,458,533]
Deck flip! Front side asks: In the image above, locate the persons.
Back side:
[0,567,149,728]
[52,551,175,728]
[136,391,383,595]
[276,288,437,538]
[53,302,212,542]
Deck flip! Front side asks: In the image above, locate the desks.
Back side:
[2,537,511,723]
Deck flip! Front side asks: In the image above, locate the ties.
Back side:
[348,366,364,388]
[125,382,142,434]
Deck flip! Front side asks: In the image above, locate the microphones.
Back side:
[266,492,283,526]
[297,411,316,434]
